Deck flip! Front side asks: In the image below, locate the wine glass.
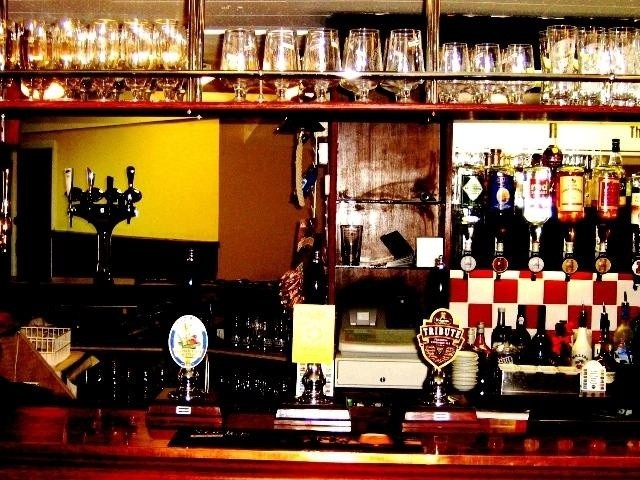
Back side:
[440,25,638,107]
[0,18,192,103]
[219,24,426,103]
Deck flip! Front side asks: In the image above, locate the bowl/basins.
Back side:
[453,352,480,392]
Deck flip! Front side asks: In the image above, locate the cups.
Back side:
[339,224,365,265]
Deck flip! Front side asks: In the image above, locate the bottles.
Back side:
[458,291,637,367]
[226,314,291,355]
[450,122,640,292]
